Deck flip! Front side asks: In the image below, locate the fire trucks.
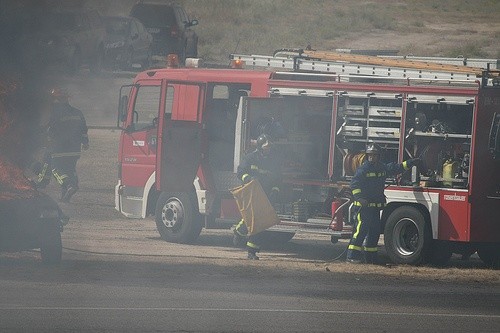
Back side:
[114,49,499,264]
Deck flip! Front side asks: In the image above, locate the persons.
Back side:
[48,86,90,200]
[233,134,283,260]
[25,153,50,188]
[345,143,423,267]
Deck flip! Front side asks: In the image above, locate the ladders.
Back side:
[227,49,500,87]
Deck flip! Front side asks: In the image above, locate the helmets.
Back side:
[257,133,273,150]
[365,143,382,155]
[48,84,71,98]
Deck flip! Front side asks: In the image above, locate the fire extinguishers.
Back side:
[331,195,343,231]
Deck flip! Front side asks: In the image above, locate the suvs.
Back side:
[129,2,198,65]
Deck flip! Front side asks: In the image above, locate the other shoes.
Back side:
[230,225,240,247]
[247,251,259,260]
[61,182,79,203]
[347,257,362,264]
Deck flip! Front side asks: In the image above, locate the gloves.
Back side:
[412,158,422,166]
[359,198,369,207]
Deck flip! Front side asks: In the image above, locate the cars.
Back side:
[0,163,68,263]
[101,15,153,73]
[43,7,101,69]
